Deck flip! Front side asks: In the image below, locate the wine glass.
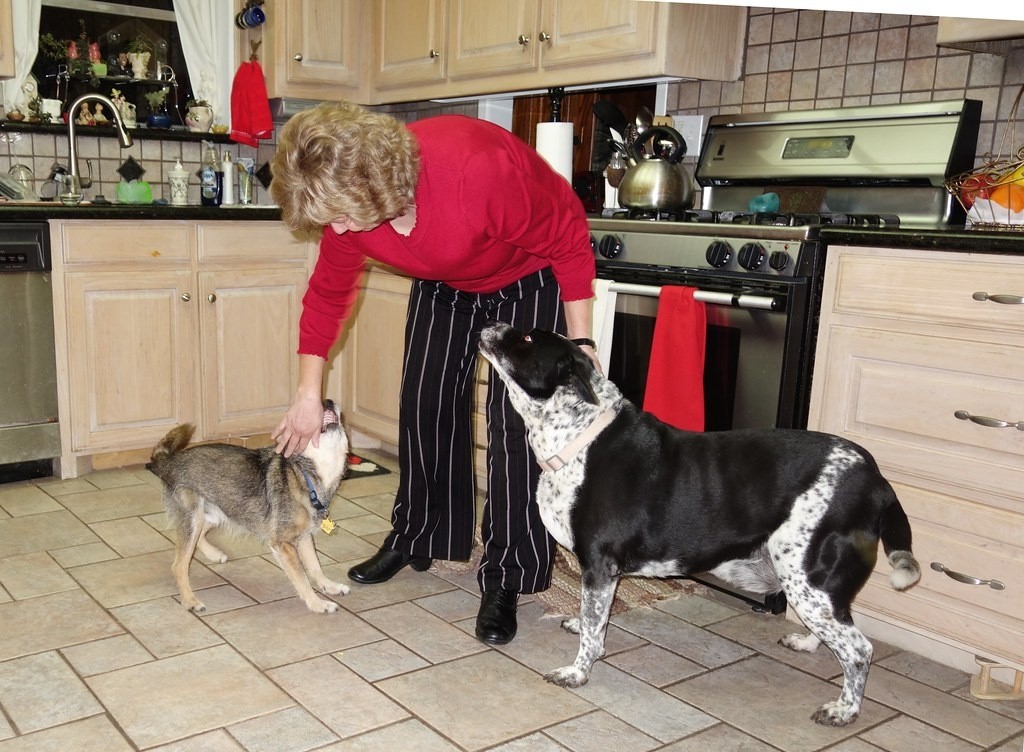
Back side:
[0,131,35,194]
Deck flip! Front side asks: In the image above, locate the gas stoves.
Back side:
[584,98,981,241]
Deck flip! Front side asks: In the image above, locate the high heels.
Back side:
[348,543,432,583]
[474,585,521,645]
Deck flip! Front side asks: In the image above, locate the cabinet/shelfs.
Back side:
[373,0,447,105]
[344,261,490,496]
[46,219,309,480]
[785,243,1024,688]
[448,0,746,97]
[2,34,240,145]
[235,0,373,104]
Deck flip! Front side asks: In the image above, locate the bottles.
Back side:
[200,141,223,206]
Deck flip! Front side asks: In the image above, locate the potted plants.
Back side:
[127,35,153,80]
[184,98,215,133]
[142,87,173,130]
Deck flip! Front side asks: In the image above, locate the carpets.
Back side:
[339,452,391,481]
[427,520,686,619]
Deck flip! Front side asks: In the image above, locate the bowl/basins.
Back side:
[211,124,229,134]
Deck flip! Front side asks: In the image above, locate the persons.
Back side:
[270,103,602,645]
[93,103,110,121]
[77,102,96,125]
[110,88,136,119]
[112,52,134,77]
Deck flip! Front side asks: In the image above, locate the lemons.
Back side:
[997,166,1024,186]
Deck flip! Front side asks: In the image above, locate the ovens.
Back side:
[572,223,826,604]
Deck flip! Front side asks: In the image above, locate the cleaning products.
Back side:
[199,139,256,207]
[167,157,191,206]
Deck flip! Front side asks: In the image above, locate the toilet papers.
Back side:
[536,122,575,188]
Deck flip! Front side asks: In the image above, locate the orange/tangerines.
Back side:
[989,172,1024,213]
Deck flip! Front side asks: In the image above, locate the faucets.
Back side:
[67,94,135,202]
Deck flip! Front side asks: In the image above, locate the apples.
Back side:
[960,174,994,208]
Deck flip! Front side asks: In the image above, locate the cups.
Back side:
[168,170,191,205]
[58,175,84,206]
[236,4,265,30]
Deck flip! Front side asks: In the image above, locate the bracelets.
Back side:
[571,338,595,349]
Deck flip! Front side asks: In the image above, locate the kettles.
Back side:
[617,125,696,212]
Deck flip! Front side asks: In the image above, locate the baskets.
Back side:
[940,84,1024,235]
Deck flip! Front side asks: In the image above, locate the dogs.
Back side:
[479,321,921,728]
[145,399,353,615]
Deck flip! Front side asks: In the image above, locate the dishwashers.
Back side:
[0,218,62,484]
